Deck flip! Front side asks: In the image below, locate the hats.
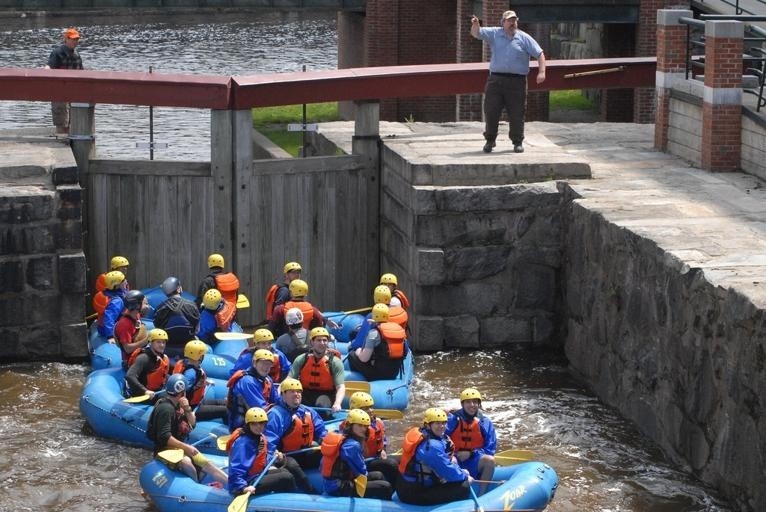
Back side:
[64,29,80,39]
[502,10,518,20]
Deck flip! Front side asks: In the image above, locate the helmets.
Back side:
[349,391,374,408]
[459,388,482,401]
[252,349,274,362]
[111,255,130,269]
[423,408,448,423]
[204,288,222,311]
[148,328,169,341]
[284,261,302,273]
[124,289,144,310]
[166,373,187,395]
[285,307,304,325]
[104,270,126,291]
[308,326,329,341]
[207,253,225,269]
[345,408,372,426]
[279,379,303,392]
[245,407,269,424]
[162,276,179,294]
[254,328,274,343]
[370,272,398,324]
[288,279,309,297]
[184,340,206,361]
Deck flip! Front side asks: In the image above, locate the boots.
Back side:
[299,477,322,494]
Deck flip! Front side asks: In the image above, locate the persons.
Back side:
[348,273,409,382]
[45,29,81,137]
[92,252,239,361]
[400,388,496,507]
[470,11,545,153]
[319,393,400,497]
[228,262,345,497]
[122,328,228,485]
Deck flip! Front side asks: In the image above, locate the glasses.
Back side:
[291,325,302,328]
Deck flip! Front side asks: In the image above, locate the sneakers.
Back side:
[514,145,523,153]
[483,142,496,152]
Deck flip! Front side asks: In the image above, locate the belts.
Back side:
[491,71,526,78]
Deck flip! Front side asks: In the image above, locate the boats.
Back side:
[89,287,251,379]
[80,366,382,448]
[322,307,410,410]
[140,448,557,512]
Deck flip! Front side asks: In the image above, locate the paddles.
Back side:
[343,380,371,398]
[216,434,231,451]
[305,405,404,421]
[227,452,278,512]
[491,449,539,467]
[156,432,218,465]
[121,389,166,404]
[213,332,254,342]
[363,447,405,464]
[235,293,251,309]
[353,473,368,498]
